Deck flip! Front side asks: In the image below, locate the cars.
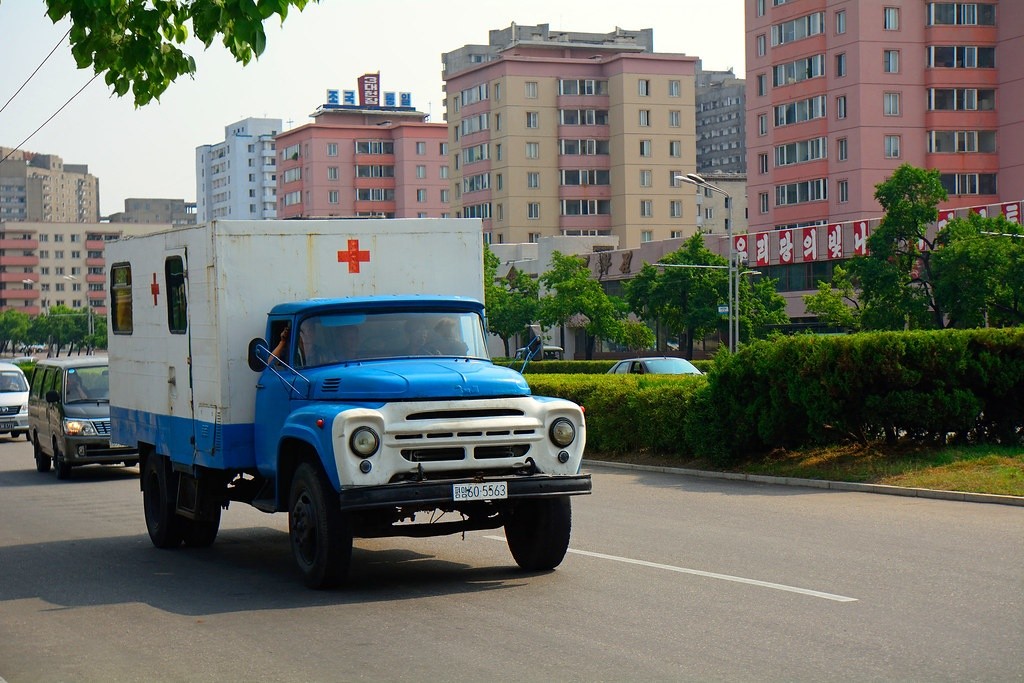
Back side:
[603,356,709,376]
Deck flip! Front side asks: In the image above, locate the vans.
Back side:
[27,355,140,480]
[0,362,36,438]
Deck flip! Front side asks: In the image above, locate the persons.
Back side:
[23,345,30,357]
[639,363,644,374]
[399,317,470,357]
[335,322,368,362]
[59,369,88,400]
[1,374,12,389]
[266,318,339,370]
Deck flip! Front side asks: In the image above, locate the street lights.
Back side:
[674,172,734,358]
[23,278,54,358]
[63,275,92,356]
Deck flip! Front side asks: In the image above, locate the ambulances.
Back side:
[101,215,594,590]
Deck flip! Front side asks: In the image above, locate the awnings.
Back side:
[772,281,947,318]
